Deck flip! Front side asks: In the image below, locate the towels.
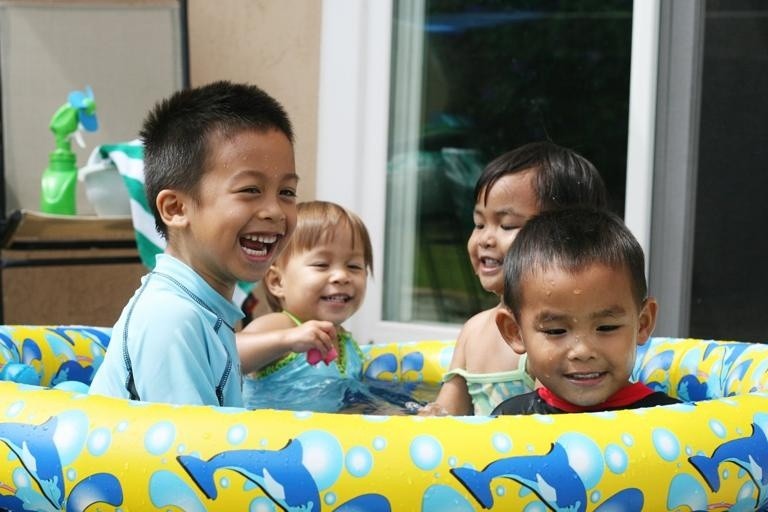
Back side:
[88,134,261,315]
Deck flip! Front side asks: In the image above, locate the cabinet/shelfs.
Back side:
[0,208,148,326]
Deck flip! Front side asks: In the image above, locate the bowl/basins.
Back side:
[78,158,130,216]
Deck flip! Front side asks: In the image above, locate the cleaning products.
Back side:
[39,97,98,214]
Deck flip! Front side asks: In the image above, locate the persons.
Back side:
[90,81,299,409]
[235,200,375,413]
[415,141,608,417]
[491,207,683,414]
